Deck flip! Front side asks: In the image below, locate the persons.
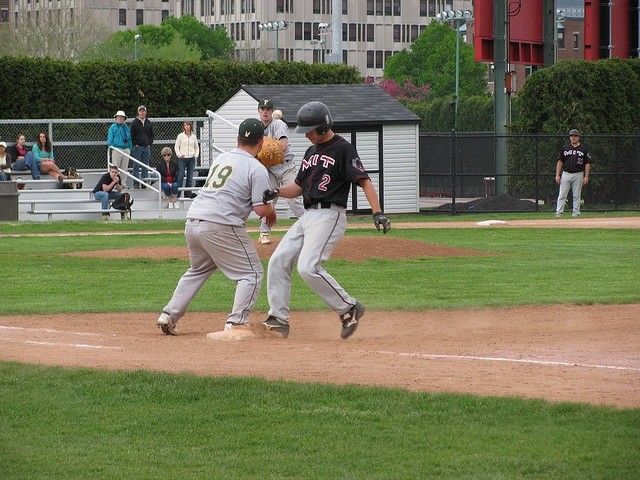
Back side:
[157,147,181,209]
[32,131,70,185]
[11,134,41,179]
[92,165,124,219]
[555,129,590,216]
[156,117,276,333]
[0,141,11,181]
[174,121,200,197]
[262,102,391,339]
[107,111,132,189]
[256,99,302,245]
[130,104,154,189]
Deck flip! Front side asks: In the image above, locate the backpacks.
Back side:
[109,193,130,210]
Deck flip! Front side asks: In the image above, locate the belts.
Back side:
[308,203,331,209]
[565,170,581,173]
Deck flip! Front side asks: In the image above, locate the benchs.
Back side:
[179,198,195,209]
[4,169,68,179]
[178,186,204,193]
[143,177,209,183]
[20,197,117,210]
[28,208,137,221]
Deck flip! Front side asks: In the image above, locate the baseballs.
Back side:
[272,109,283,119]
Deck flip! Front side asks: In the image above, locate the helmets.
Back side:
[295,101,333,134]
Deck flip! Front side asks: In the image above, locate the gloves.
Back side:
[373,213,390,234]
[262,188,279,205]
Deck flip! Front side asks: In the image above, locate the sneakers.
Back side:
[174,203,180,209]
[339,302,364,339]
[23,166,28,171]
[225,324,248,332]
[157,312,179,334]
[169,202,173,209]
[260,315,289,338]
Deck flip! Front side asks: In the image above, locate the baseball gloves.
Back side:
[256,137,285,166]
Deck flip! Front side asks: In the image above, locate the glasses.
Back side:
[162,154,171,156]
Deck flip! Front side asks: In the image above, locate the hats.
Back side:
[259,100,272,108]
[138,105,146,110]
[0,142,7,148]
[239,118,264,138]
[114,111,126,117]
[569,129,580,136]
